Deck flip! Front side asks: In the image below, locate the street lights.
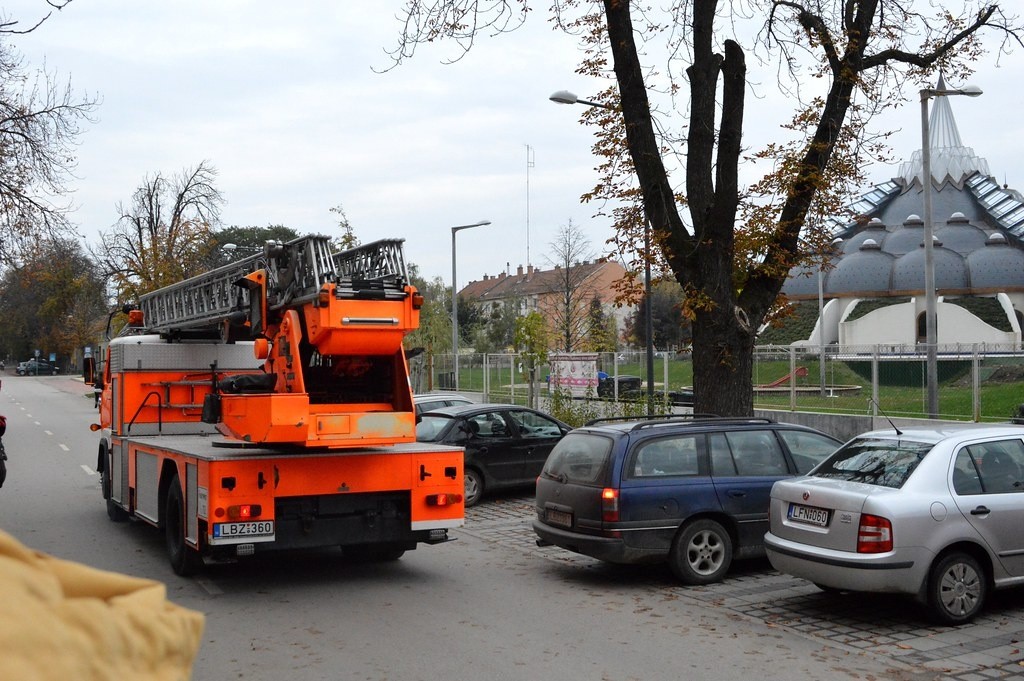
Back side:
[919,85,986,421]
[450,219,492,391]
[550,89,658,421]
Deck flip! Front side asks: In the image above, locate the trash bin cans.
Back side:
[599,375,643,397]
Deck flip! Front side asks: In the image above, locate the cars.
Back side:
[414,404,594,507]
[411,395,476,424]
[762,423,1024,625]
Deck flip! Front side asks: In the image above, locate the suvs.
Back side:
[16,359,61,376]
[530,413,851,590]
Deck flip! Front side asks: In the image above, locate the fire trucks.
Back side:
[81,231,466,582]
